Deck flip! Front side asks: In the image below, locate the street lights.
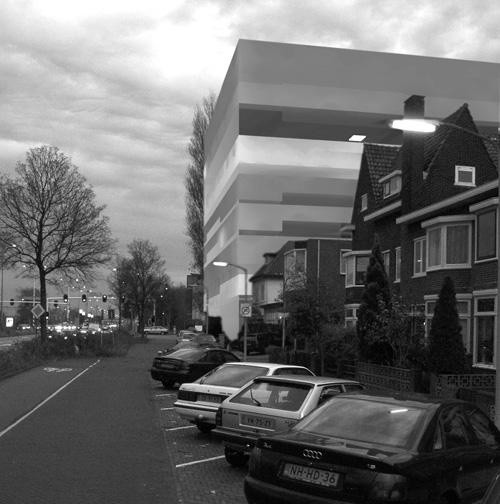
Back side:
[23,265,46,326]
[60,279,78,322]
[0,243,16,311]
[387,117,500,145]
[213,260,248,362]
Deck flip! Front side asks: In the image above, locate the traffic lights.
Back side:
[82,294,86,302]
[102,295,107,302]
[63,294,68,303]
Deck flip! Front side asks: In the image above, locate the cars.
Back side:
[212,375,370,465]
[143,325,242,388]
[174,362,318,433]
[243,388,500,503]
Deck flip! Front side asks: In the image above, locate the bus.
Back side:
[99,319,123,330]
